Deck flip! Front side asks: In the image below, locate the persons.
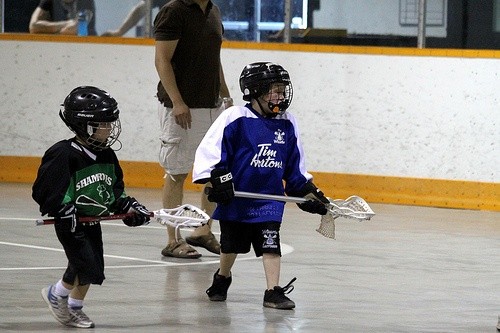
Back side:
[154,0,233,257]
[30,0,169,37]
[32,86,148,328]
[192,62,331,308]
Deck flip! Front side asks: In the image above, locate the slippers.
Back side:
[186,232,221,253]
[161,238,202,258]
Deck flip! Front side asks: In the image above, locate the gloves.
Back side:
[114,197,151,226]
[208,166,237,204]
[57,203,86,240]
[296,178,331,215]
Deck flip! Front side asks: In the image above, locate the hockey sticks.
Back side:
[34,204,209,242]
[204,186,375,222]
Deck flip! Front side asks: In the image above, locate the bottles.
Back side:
[77,13,87,36]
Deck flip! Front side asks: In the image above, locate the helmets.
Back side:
[239,62,291,101]
[59,86,119,138]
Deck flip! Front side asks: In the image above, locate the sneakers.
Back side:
[206,268,233,301]
[263,277,298,308]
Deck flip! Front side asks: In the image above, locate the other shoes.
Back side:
[47,288,72,323]
[58,306,95,328]
